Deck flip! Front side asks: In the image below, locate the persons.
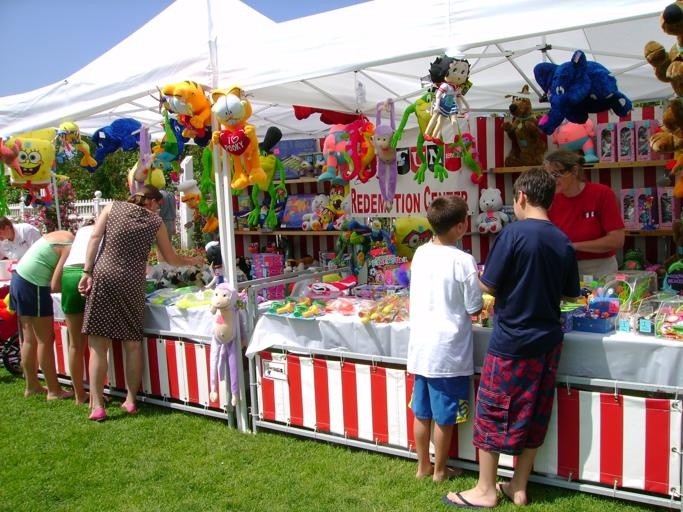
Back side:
[77,185,205,421]
[545,150,625,279]
[408,194,482,480]
[0,216,42,263]
[10,221,77,401]
[442,167,581,508]
[51,219,111,403]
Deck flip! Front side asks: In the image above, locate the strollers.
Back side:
[0,285,21,375]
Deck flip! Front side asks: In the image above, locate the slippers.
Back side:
[22,384,48,400]
[495,481,528,507]
[42,387,74,402]
[71,389,110,405]
[441,487,498,509]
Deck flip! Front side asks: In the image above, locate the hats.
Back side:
[205,245,222,266]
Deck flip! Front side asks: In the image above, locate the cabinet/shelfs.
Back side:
[487,158,676,262]
[233,178,347,253]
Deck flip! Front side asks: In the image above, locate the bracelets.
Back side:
[82,269,90,274]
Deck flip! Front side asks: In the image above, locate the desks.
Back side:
[241,297,681,392]
[51,291,218,334]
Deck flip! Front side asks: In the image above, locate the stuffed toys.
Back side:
[643,0,683,97]
[533,50,632,135]
[649,101,683,198]
[474,187,508,234]
[151,82,289,231]
[388,53,471,182]
[300,183,397,272]
[87,116,143,173]
[314,99,398,201]
[500,85,548,167]
[1,121,97,207]
[157,241,254,292]
[601,127,674,223]
[208,283,247,406]
[128,53,470,406]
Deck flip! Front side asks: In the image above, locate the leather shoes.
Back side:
[86,406,108,422]
[120,400,140,415]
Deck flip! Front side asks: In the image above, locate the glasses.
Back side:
[549,168,572,179]
